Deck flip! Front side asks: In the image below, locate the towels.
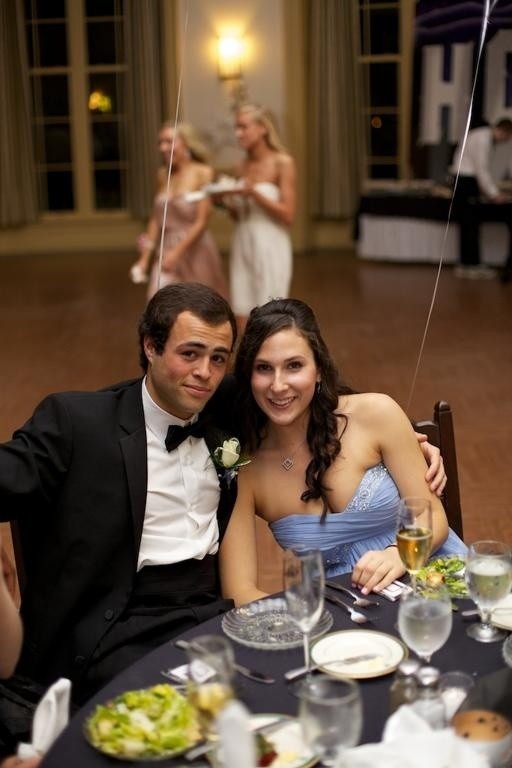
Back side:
[19,677,74,763]
[338,705,493,768]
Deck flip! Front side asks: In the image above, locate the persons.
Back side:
[1,283,448,714]
[219,298,469,610]
[445,119,512,281]
[129,121,228,308]
[208,101,298,346]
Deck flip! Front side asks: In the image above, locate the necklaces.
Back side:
[271,428,306,471]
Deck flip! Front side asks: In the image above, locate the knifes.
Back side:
[174,637,277,686]
[283,648,382,681]
[185,716,297,762]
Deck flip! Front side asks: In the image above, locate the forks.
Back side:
[309,586,373,631]
[325,577,385,611]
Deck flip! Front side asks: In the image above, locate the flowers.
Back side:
[202,432,257,489]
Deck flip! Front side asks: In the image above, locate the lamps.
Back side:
[202,21,251,80]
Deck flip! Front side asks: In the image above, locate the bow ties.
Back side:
[164,419,210,453]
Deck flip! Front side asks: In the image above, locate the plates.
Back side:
[308,628,408,679]
[82,684,205,766]
[203,711,322,768]
[220,597,336,654]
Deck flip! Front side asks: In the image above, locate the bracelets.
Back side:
[384,544,399,550]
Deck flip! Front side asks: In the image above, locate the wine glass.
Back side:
[185,637,235,687]
[293,674,365,768]
[395,497,434,599]
[283,546,324,675]
[466,539,512,646]
[398,582,454,668]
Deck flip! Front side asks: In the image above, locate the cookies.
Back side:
[452,709,510,745]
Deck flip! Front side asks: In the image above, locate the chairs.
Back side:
[411,401,464,544]
